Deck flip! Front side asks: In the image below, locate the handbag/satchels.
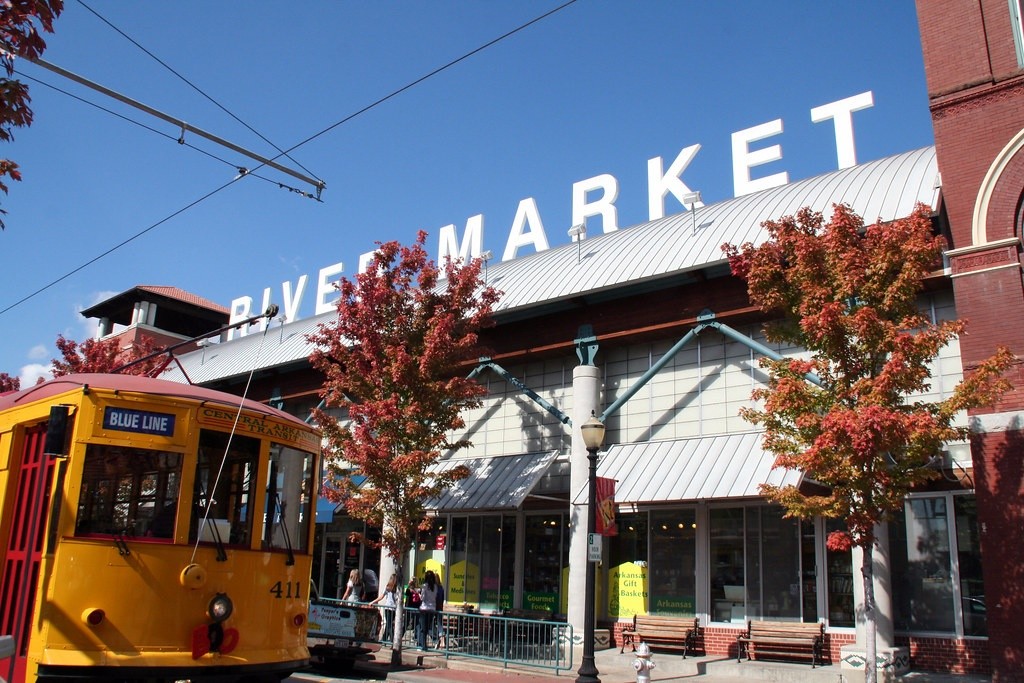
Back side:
[359,582,364,597]
[412,592,420,602]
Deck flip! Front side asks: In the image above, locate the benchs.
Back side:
[736,618,826,669]
[620,614,700,660]
[439,604,474,644]
[489,608,554,644]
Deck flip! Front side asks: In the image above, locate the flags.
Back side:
[595,477,619,536]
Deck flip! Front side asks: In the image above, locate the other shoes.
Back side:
[378,640,386,646]
[439,642,446,647]
[416,646,428,652]
[434,640,440,650]
[410,638,416,643]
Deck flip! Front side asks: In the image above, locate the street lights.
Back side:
[575,408,607,683]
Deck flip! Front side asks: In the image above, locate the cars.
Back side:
[908,573,988,639]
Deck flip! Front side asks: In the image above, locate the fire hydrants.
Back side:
[632,641,656,683]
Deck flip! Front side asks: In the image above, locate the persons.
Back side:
[340,568,365,606]
[369,573,399,642]
[404,571,446,651]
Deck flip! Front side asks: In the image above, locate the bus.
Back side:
[0,370,326,683]
